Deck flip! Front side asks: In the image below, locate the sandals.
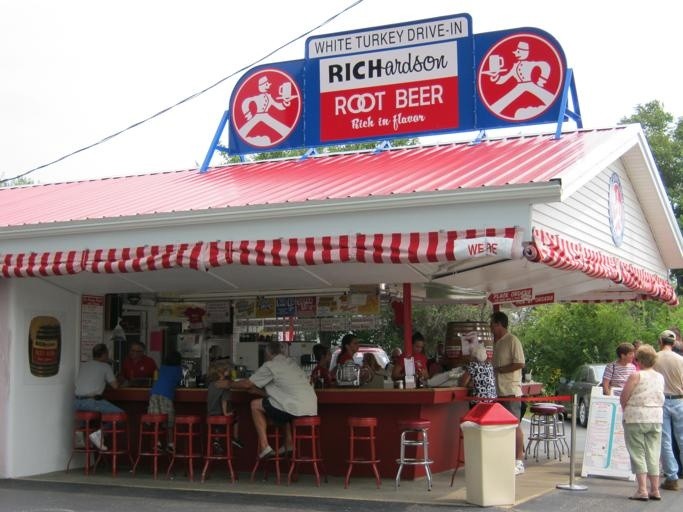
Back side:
[649,490,661,500]
[628,490,649,501]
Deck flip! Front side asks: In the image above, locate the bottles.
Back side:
[312,369,325,391]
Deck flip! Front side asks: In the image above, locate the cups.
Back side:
[525,373,530,383]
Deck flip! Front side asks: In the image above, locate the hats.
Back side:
[659,330,676,341]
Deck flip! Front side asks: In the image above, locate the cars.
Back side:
[332,345,390,370]
[555,364,607,427]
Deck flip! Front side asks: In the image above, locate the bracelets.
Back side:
[229,380,234,388]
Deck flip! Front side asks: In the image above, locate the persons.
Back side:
[457,344,498,411]
[652,329,683,492]
[74,344,119,450]
[208,345,233,376]
[488,311,525,477]
[603,342,635,396]
[667,326,683,345]
[308,331,454,390]
[620,343,666,501]
[207,362,245,451]
[215,341,318,460]
[147,351,183,454]
[115,341,155,389]
[672,340,683,354]
[632,339,645,371]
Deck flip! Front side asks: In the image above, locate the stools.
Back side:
[450,416,467,487]
[66,410,329,488]
[524,403,571,463]
[343,415,383,490]
[393,418,436,493]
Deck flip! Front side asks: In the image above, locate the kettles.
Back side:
[335,359,360,389]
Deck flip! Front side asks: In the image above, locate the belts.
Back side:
[76,395,103,400]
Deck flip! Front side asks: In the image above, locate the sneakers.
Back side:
[89,429,108,452]
[661,478,679,491]
[152,441,162,453]
[166,442,175,454]
[75,431,86,449]
[515,460,525,475]
[257,446,273,460]
[214,440,224,452]
[232,438,245,449]
[279,445,292,456]
